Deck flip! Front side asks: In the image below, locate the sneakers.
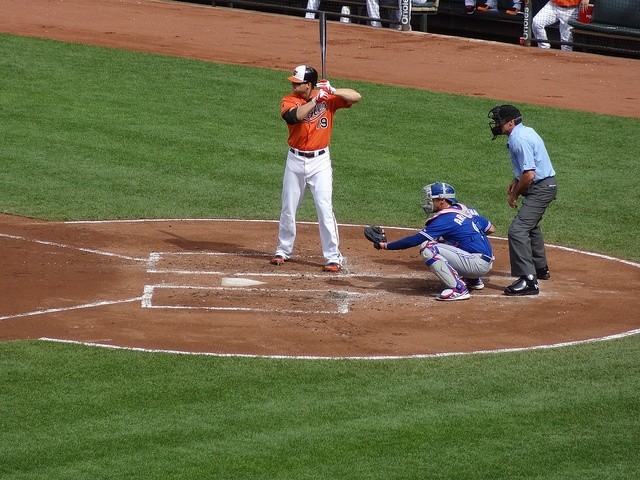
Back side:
[535,264,550,280]
[504,274,539,296]
[465,4,477,14]
[459,277,485,290]
[435,286,471,301]
[477,3,498,12]
[270,251,286,265]
[323,257,344,272]
[506,5,522,15]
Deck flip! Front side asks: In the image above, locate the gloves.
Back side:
[315,79,336,95]
[311,89,329,106]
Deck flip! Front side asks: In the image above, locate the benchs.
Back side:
[568,1,640,59]
[328,0,439,33]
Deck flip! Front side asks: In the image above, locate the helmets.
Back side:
[288,64,319,87]
[488,105,521,140]
[419,181,459,214]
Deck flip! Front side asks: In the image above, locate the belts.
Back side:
[289,148,325,159]
[558,5,577,9]
[480,253,494,262]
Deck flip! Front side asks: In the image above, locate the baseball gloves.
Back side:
[364,226,387,250]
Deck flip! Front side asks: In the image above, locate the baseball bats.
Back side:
[319,13,326,80]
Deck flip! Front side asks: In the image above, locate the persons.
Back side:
[487,105,557,296]
[366,0,427,31]
[364,182,496,301]
[304,0,352,24]
[270,65,362,272]
[532,0,590,52]
[476,0,522,16]
